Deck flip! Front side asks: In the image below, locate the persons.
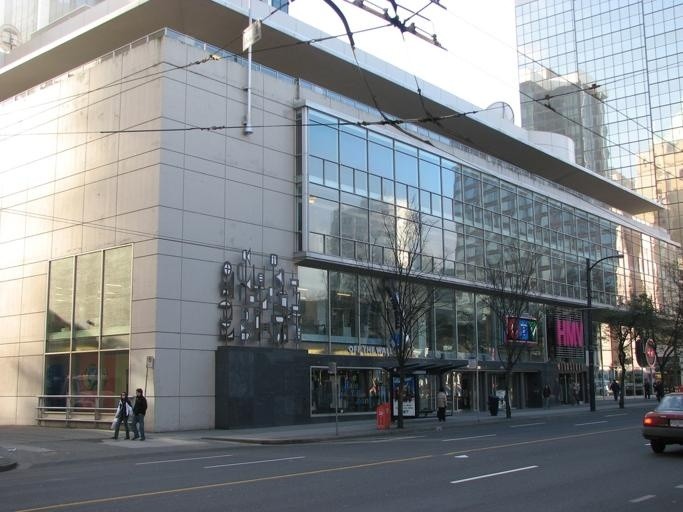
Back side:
[656,380,664,398]
[540,383,550,410]
[129,388,147,441]
[643,382,651,399]
[435,385,447,422]
[609,379,619,401]
[107,391,130,441]
[652,383,661,402]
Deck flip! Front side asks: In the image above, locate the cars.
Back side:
[641,391,683,454]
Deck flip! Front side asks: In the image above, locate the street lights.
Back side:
[583,254,626,414]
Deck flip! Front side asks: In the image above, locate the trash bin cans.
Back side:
[488,395,499,416]
[376,403,391,430]
